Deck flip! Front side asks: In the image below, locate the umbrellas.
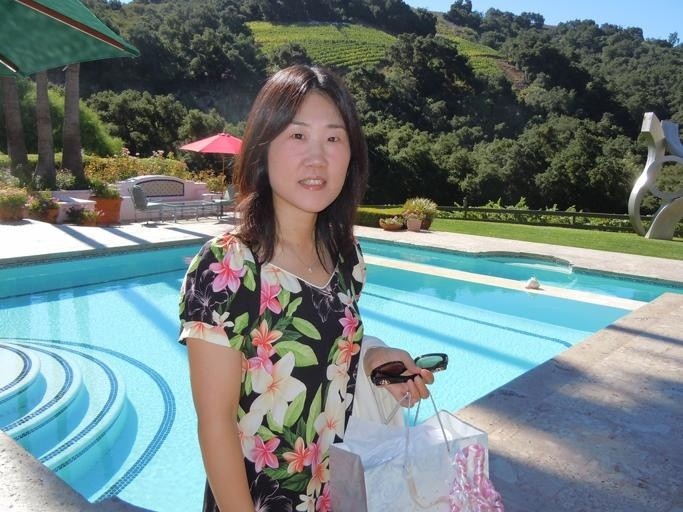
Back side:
[178,130,246,200]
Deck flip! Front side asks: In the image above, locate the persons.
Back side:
[180,63,434,512]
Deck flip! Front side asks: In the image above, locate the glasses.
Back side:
[370,353,449,387]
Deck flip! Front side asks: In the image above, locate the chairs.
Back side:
[128,185,164,225]
[212,184,234,221]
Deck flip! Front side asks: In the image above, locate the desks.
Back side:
[161,200,215,223]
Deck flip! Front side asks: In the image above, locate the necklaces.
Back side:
[284,242,322,273]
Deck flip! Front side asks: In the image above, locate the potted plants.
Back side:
[378,195,437,232]
[89,178,124,226]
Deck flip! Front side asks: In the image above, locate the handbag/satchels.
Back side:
[329,388,506,512]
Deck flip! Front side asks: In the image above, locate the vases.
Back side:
[0,205,25,221]
[30,208,59,223]
[82,218,96,226]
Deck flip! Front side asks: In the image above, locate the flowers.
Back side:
[25,188,60,217]
[0,186,31,212]
[81,205,104,225]
